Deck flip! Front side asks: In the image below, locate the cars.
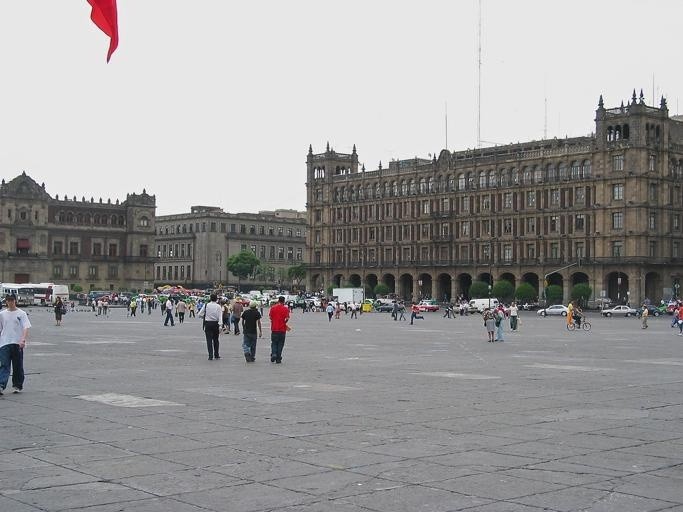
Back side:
[601,305,637,317]
[137,286,405,312]
[411,300,440,312]
[537,304,568,317]
[445,303,478,313]
[635,305,663,317]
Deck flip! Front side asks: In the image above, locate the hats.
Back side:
[5,294,16,300]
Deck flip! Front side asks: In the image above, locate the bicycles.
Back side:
[567,315,591,331]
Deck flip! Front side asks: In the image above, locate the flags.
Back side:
[87,1,123,66]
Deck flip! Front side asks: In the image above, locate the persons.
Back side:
[240,302,262,362]
[268,296,289,363]
[520,296,683,335]
[368,291,519,342]
[0,292,32,395]
[78,286,210,326]
[53,296,76,326]
[215,287,363,336]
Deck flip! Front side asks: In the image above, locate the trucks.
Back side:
[468,298,499,314]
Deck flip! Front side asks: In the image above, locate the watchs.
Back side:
[197,294,223,361]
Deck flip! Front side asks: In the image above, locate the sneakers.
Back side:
[12,387,20,393]
[271,354,281,363]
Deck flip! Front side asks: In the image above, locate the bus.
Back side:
[0,283,35,305]
[25,283,69,306]
[89,291,133,304]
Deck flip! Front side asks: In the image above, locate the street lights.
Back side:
[488,284,492,311]
[483,245,492,283]
[612,245,622,304]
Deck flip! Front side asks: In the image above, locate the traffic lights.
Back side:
[578,258,584,268]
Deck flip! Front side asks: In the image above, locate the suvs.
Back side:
[517,297,539,311]
